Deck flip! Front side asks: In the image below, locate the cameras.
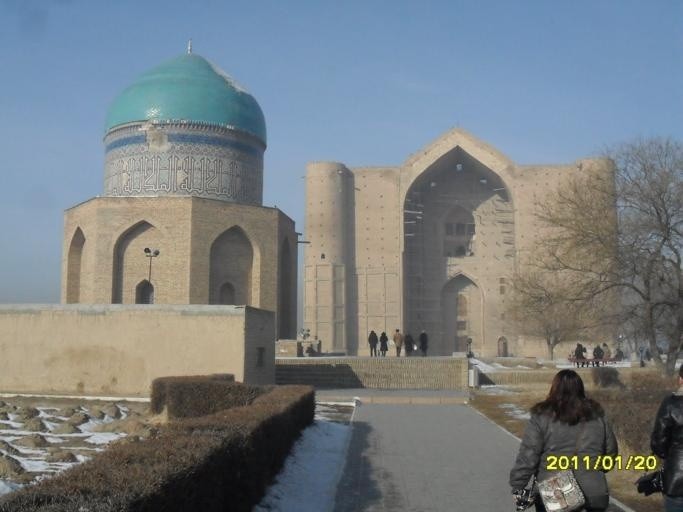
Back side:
[633,470,665,497]
[513,488,530,512]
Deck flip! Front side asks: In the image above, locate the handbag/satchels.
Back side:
[537,468,585,512]
[634,471,663,496]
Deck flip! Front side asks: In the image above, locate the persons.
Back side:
[646,348,652,361]
[510,369,618,511]
[297,328,304,357]
[615,348,625,364]
[405,331,418,356]
[418,329,427,357]
[380,332,388,356]
[649,362,683,512]
[393,329,405,356]
[601,343,611,366]
[368,331,379,357]
[575,342,586,367]
[592,344,604,367]
[303,328,310,340]
